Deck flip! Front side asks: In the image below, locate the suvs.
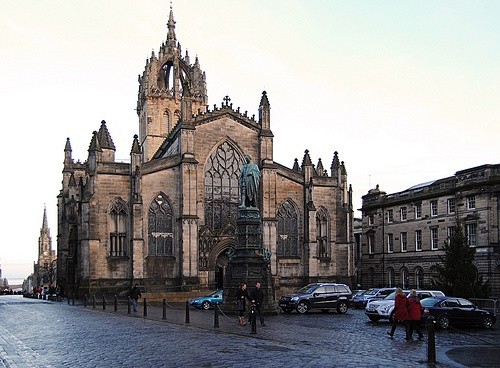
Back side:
[365,289,446,325]
[277,283,354,315]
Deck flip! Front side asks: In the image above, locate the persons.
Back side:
[387,288,424,341]
[249,281,267,327]
[129,284,141,312]
[1,284,63,305]
[234,154,261,209]
[236,283,254,326]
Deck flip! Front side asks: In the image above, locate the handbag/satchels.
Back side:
[234,300,243,311]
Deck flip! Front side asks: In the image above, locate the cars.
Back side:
[190,289,224,310]
[351,288,397,308]
[350,290,367,302]
[420,296,497,330]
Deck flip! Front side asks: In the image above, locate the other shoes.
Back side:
[387,332,393,337]
[404,338,409,340]
[261,321,264,326]
[417,336,424,339]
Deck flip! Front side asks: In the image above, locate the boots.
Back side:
[239,317,247,326]
